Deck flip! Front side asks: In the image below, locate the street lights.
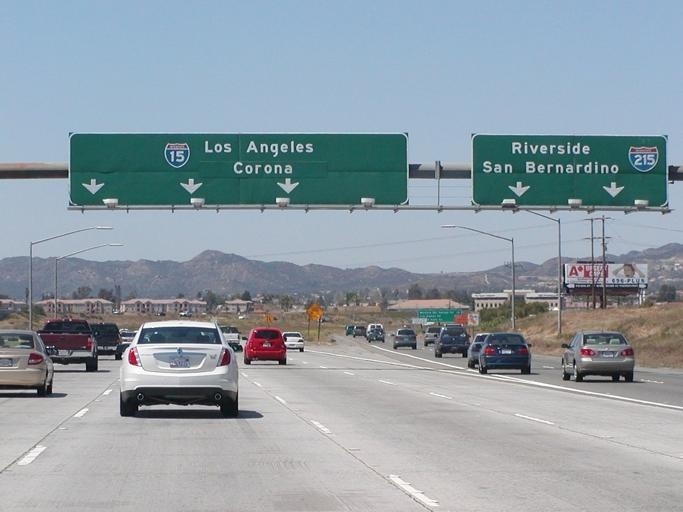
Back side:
[28,225,113,333]
[51,243,128,318]
[436,223,515,332]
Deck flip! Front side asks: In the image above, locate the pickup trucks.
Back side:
[179,311,192,317]
[33,318,100,372]
[87,322,122,360]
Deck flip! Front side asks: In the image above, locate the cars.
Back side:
[238,314,249,320]
[156,311,165,316]
[114,309,124,314]
[477,333,531,374]
[465,332,492,368]
[341,320,389,345]
[281,331,304,353]
[0,328,55,397]
[241,327,287,367]
[559,329,636,382]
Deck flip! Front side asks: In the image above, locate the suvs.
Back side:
[114,318,242,419]
[432,324,471,358]
[390,327,418,350]
[421,326,440,347]
[116,324,243,358]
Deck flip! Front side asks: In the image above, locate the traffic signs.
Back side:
[417,307,464,324]
[66,131,409,210]
[468,132,668,213]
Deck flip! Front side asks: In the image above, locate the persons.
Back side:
[611,263,645,279]
